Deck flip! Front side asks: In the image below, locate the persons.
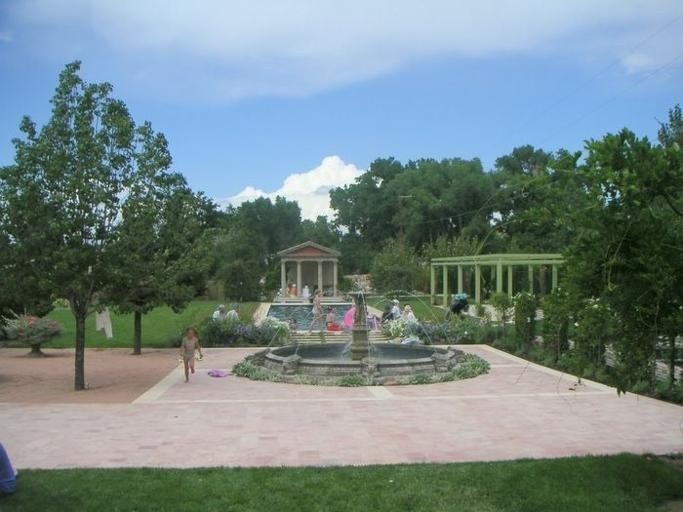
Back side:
[285,279,317,297]
[212,303,225,323]
[306,288,325,335]
[342,299,413,332]
[288,317,297,329]
[178,327,203,383]
[224,303,240,321]
[325,305,343,331]
